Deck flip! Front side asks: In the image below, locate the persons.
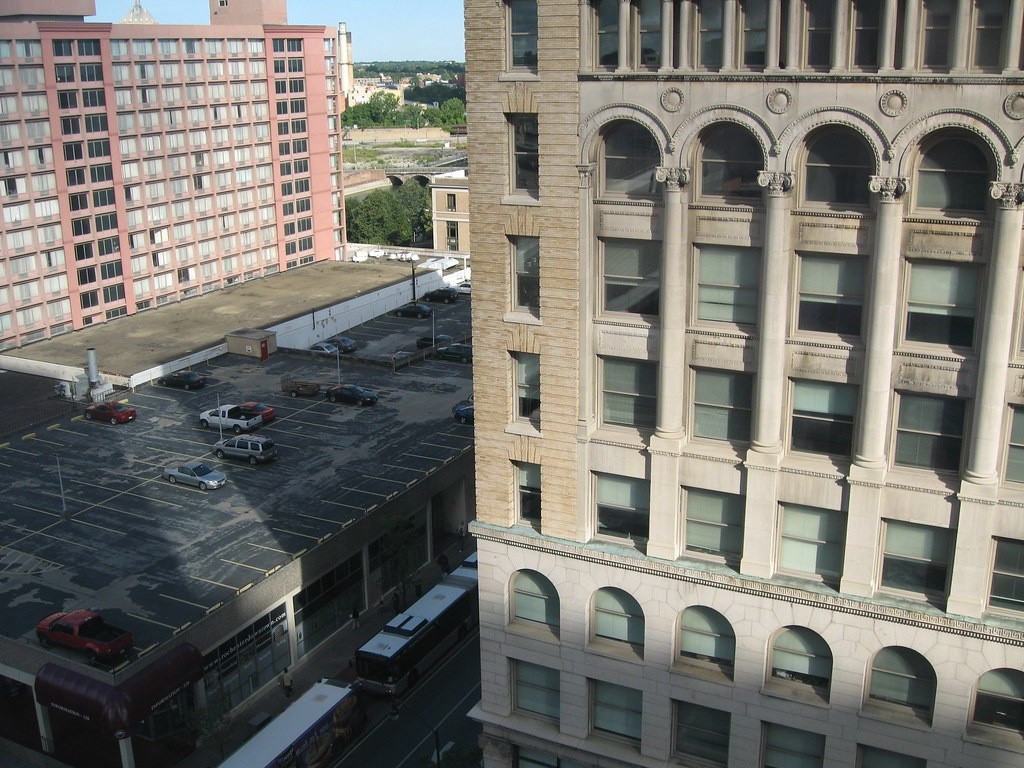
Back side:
[377,521,466,617]
[282,668,293,700]
[352,605,361,631]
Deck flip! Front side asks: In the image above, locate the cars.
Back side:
[308,341,340,358]
[395,304,433,319]
[327,385,378,407]
[436,343,473,362]
[239,401,278,421]
[157,370,208,391]
[163,460,227,490]
[85,400,137,424]
[286,382,321,398]
[450,283,472,294]
[454,392,475,425]
[327,336,358,353]
[416,335,453,350]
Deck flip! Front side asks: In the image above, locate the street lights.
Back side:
[389,694,440,768]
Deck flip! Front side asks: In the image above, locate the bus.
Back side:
[211,676,368,767]
[348,549,488,698]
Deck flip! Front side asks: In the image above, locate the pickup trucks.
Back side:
[36,611,134,665]
[200,404,264,434]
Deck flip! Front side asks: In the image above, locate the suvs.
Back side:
[215,433,278,464]
[423,287,459,304]
